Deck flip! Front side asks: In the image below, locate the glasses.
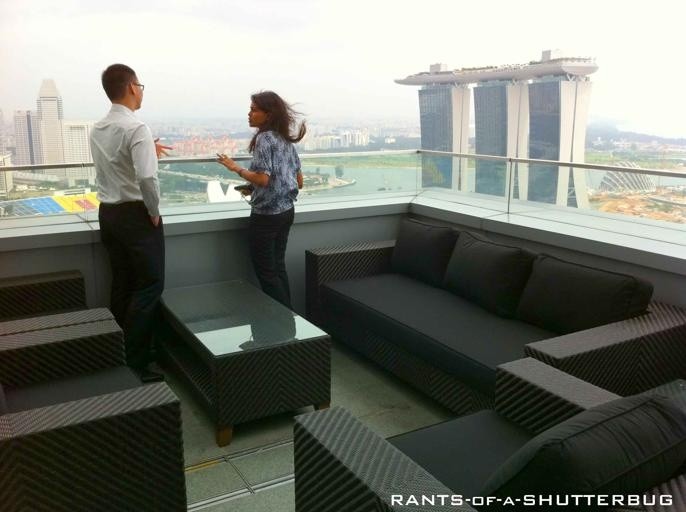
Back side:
[134,84,145,90]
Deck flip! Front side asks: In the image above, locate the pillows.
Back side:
[393,218,453,285]
[513,253,654,332]
[465,394,686,512]
[446,231,536,316]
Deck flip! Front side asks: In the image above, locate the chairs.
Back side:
[294,357,686,512]
[0,319,187,512]
[0,270,114,333]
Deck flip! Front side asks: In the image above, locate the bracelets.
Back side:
[239,168,245,176]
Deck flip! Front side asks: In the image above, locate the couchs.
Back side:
[301,238,686,415]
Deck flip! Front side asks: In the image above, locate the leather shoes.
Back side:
[139,369,165,384]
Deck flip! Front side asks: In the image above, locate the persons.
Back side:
[89,63,167,382]
[215,88,307,311]
